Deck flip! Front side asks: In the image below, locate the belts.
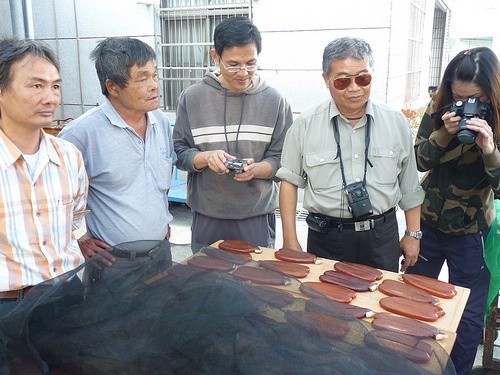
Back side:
[108,239,166,259]
[0,286,45,300]
[331,208,396,232]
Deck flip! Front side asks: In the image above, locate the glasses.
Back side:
[331,71,372,90]
[214,48,259,72]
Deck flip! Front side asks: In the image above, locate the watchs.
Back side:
[405,230,422,240]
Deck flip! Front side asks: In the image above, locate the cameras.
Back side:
[344,181,373,221]
[224,159,248,174]
[450,96,494,145]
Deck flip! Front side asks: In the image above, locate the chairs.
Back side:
[482,199,500,369]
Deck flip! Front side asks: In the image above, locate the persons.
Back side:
[57,35,177,299]
[0,37,89,374]
[414,46,500,375]
[173,17,295,257]
[275,37,425,275]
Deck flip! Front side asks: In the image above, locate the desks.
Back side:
[28,239,471,375]
